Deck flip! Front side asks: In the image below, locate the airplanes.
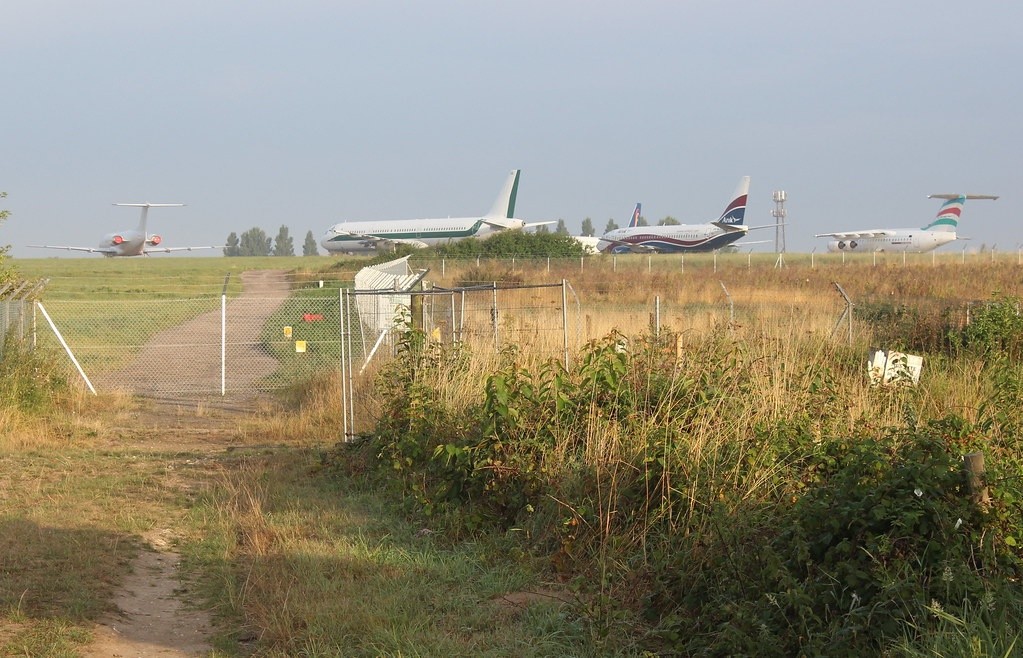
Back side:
[320,169,556,253]
[595,175,790,254]
[25,202,233,258]
[813,193,999,254]
